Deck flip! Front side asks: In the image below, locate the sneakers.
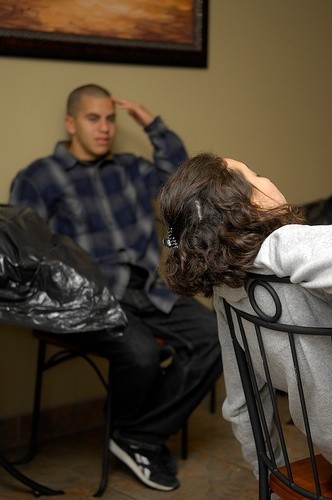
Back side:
[110,431,180,491]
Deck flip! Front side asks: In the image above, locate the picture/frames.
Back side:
[1,0,209,69]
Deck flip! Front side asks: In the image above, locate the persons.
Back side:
[9,84,224,491]
[156,151,332,483]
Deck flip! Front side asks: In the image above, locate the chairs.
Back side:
[223,263,332,500]
[0,329,216,498]
